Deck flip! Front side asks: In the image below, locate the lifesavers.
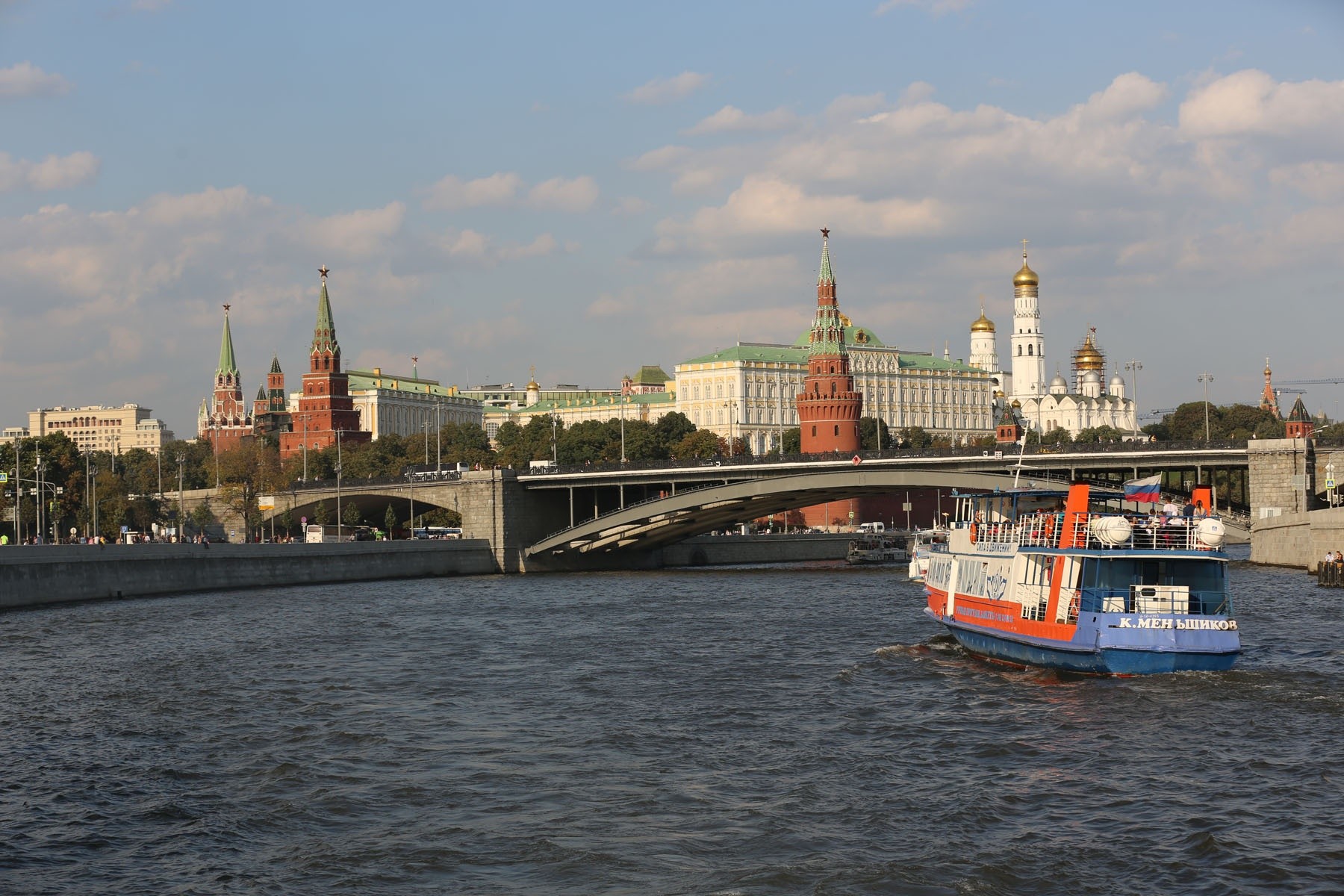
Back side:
[1071,591,1081,615]
[1044,515,1054,538]
[970,523,977,544]
[849,540,855,550]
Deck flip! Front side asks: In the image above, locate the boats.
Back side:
[844,533,912,565]
[904,421,1243,676]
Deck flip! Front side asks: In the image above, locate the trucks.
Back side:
[529,460,558,475]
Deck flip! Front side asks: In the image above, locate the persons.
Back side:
[973,496,1208,549]
[255,534,293,543]
[0,533,123,544]
[131,534,209,544]
[349,533,387,540]
[1325,551,1344,563]
[474,462,512,471]
[725,527,840,535]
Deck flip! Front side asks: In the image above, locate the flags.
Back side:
[1123,475,1161,503]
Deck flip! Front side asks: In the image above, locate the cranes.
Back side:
[1260,378,1344,399]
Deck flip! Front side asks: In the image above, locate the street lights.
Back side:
[85,443,92,539]
[154,449,166,492]
[1031,375,1050,446]
[333,428,344,480]
[213,420,223,489]
[332,461,350,544]
[107,434,120,476]
[257,437,268,490]
[421,420,432,465]
[1125,358,1145,444]
[36,440,42,538]
[41,459,57,545]
[298,412,311,483]
[175,451,188,543]
[1197,371,1215,450]
[89,464,101,538]
[619,388,630,464]
[432,401,447,480]
[1302,424,1330,512]
[724,398,738,458]
[9,437,21,544]
[549,408,561,474]
[404,465,420,540]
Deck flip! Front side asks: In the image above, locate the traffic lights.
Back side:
[49,527,54,535]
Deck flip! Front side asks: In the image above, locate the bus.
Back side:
[306,524,371,542]
[409,526,463,540]
[406,462,469,480]
[370,527,388,541]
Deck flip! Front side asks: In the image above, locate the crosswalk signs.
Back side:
[0,472,7,482]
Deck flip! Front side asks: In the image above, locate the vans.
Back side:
[857,522,885,533]
